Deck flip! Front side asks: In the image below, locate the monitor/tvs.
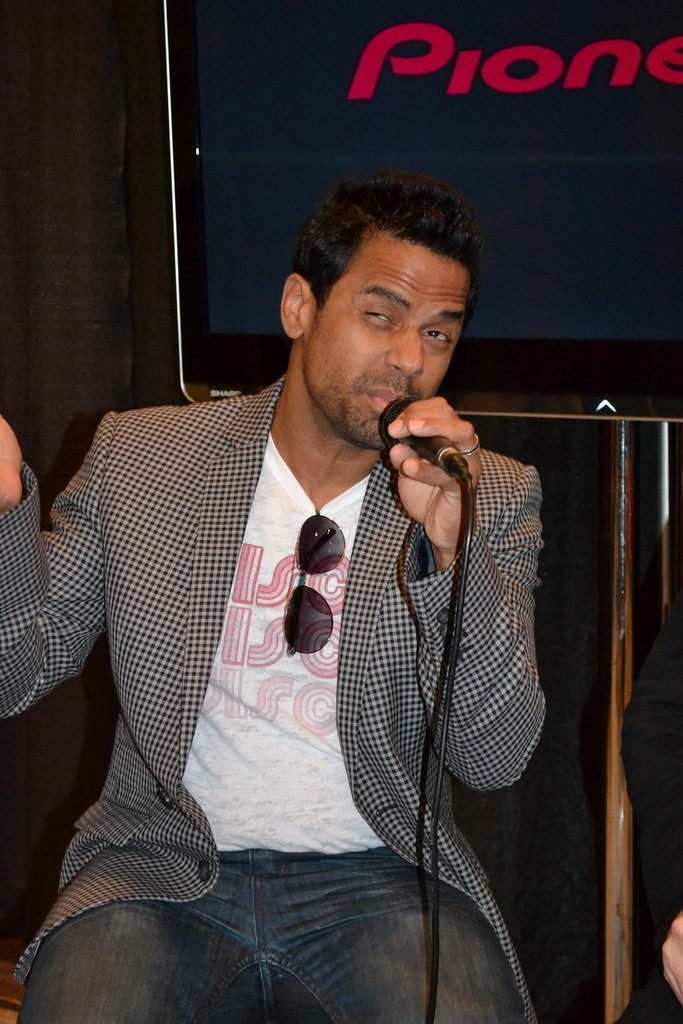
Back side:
[161,0,683,424]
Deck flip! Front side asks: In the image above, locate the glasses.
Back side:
[284,509,345,656]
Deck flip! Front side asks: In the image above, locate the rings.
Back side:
[458,433,479,456]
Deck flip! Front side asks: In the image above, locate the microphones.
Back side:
[378,394,473,486]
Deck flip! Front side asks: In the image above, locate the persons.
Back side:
[615,593,683,1024]
[0,171,547,1024]
